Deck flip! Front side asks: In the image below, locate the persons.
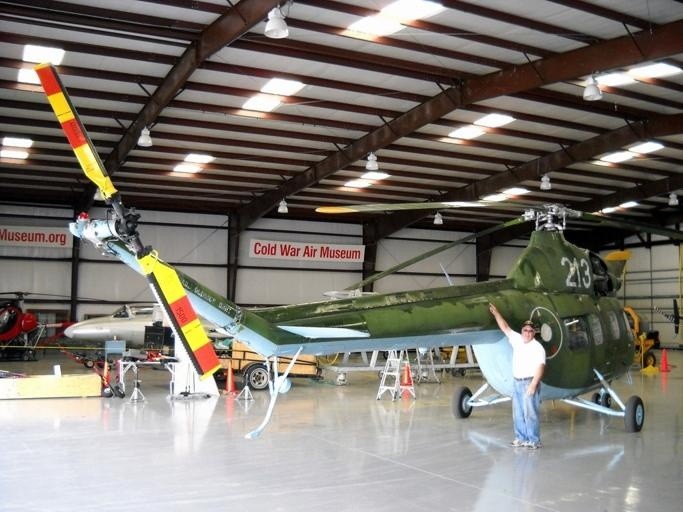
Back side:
[488,302,546,450]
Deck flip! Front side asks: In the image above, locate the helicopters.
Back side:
[33,62,662,442]
[0,291,102,362]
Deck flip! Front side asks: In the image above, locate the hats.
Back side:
[519,320,535,331]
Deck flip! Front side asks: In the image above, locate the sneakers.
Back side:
[521,441,542,449]
[508,440,523,448]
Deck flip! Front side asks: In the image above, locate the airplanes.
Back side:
[63,304,239,370]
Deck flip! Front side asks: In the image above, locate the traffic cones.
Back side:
[659,349,671,372]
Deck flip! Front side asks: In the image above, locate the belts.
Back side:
[513,376,534,382]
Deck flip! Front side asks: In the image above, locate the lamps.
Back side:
[135,120,159,148]
[262,2,290,40]
[582,71,602,102]
